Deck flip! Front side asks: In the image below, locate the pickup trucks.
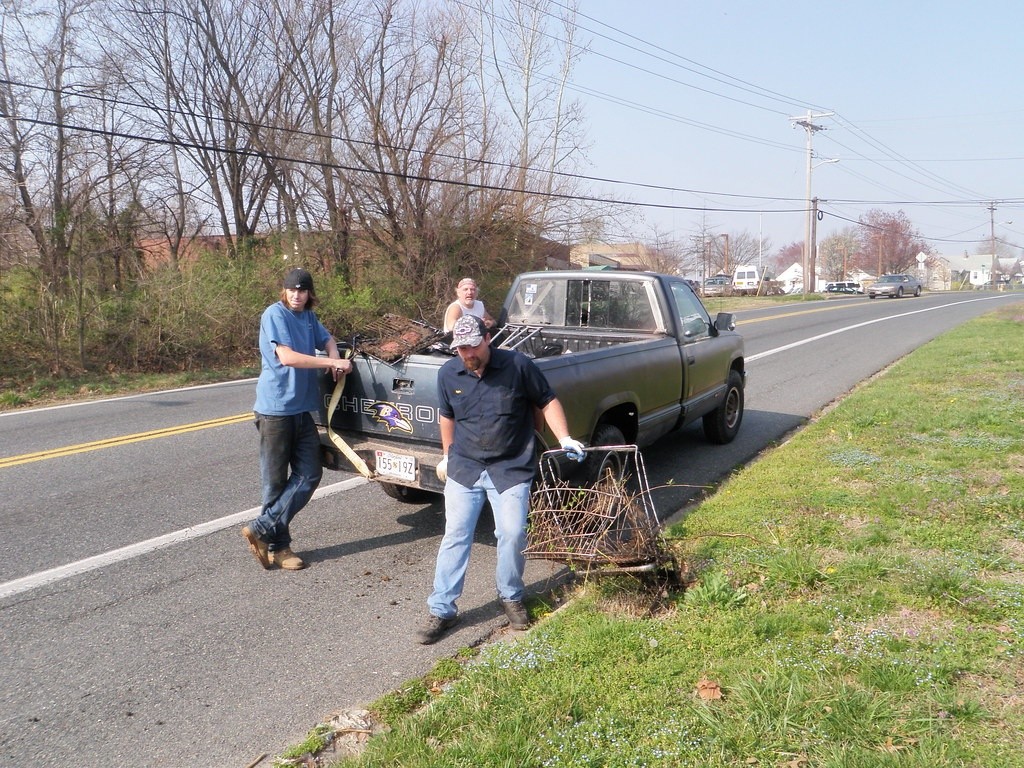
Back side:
[309,266,748,503]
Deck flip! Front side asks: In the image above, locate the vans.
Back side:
[733,265,774,297]
[824,282,864,293]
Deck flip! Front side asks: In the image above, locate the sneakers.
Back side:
[242,525,270,570]
[267,547,303,570]
[498,593,529,630]
[416,612,458,645]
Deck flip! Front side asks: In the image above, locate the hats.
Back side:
[284,268,312,290]
[450,314,486,349]
[457,278,476,287]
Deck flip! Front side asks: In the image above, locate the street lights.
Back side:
[803,159,842,297]
[992,220,1012,291]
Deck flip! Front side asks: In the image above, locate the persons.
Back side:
[444,278,497,332]
[242,268,352,570]
[415,315,588,644]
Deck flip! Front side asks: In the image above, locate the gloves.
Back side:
[560,436,587,463]
[435,456,448,482]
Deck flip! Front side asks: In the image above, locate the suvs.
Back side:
[976,280,1011,292]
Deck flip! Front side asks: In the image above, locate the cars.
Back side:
[828,286,864,295]
[703,278,734,298]
[868,274,921,299]
